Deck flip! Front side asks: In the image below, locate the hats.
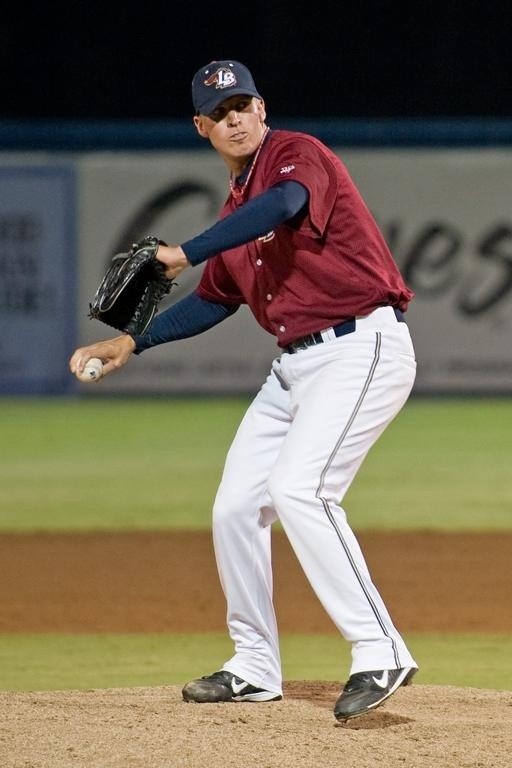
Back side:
[189,58,262,109]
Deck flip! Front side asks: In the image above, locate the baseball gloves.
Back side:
[90,236,175,336]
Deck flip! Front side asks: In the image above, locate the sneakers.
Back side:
[183,668,282,702]
[334,664,417,720]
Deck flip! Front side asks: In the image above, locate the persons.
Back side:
[67,58,417,722]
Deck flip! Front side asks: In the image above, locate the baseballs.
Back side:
[76,359,102,383]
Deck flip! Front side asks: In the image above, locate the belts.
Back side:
[263,310,407,355]
